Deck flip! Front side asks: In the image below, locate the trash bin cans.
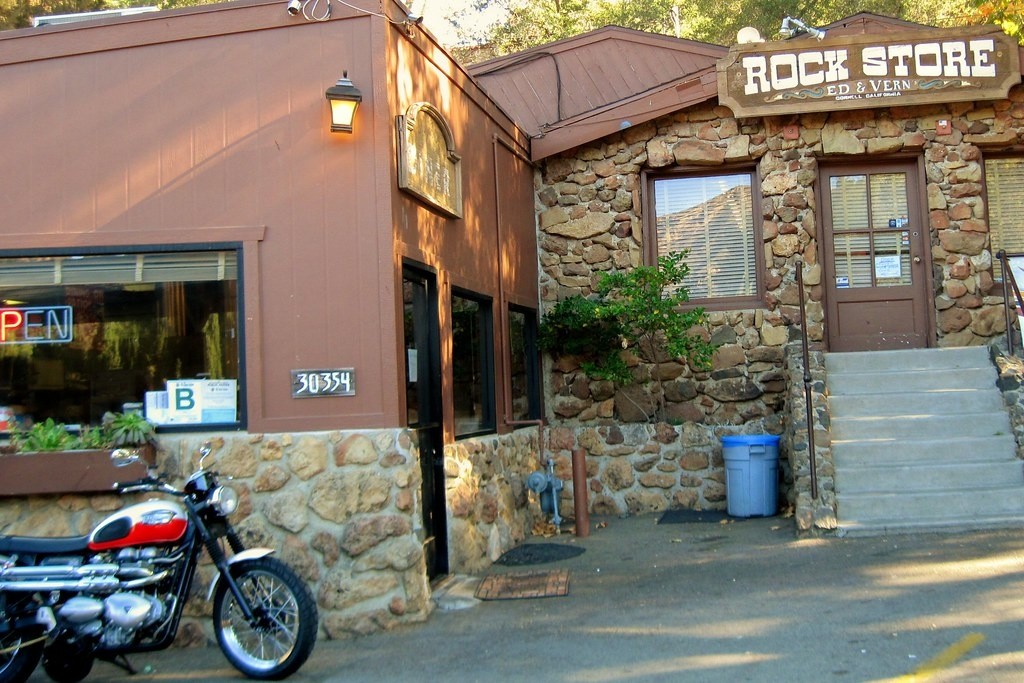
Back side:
[719,434,780,518]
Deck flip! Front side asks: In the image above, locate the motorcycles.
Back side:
[0,440,318,683]
